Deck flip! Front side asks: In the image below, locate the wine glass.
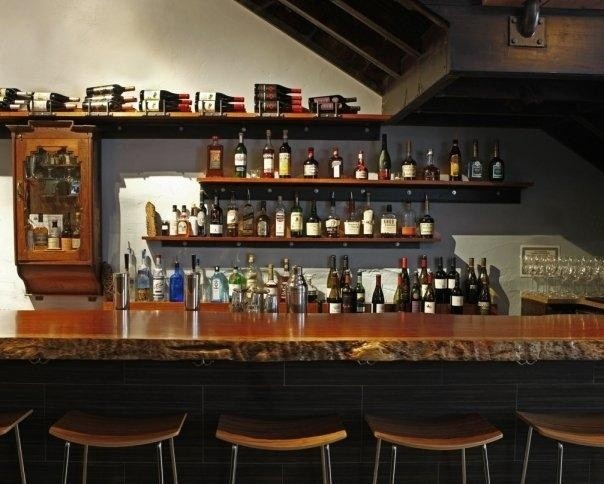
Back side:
[24,153,78,177]
[520,253,603,297]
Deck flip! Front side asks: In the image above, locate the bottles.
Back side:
[70,180,80,195]
[199,128,504,187]
[71,212,80,247]
[109,244,502,311]
[32,211,47,249]
[143,191,436,241]
[25,215,33,248]
[47,216,60,248]
[0,84,360,114]
[61,211,73,249]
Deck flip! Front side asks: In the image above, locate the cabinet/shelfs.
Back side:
[9,113,105,307]
[1,109,535,246]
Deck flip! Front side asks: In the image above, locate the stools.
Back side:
[515,403,604,484]
[1,395,38,484]
[47,408,189,484]
[359,407,503,484]
[210,409,348,484]
